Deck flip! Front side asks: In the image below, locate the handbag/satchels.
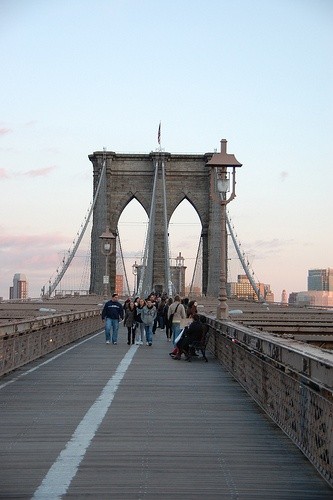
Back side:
[168,314,174,327]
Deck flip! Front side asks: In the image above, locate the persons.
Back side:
[122,291,205,361]
[101,293,124,344]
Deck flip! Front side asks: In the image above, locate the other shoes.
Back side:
[146,342,152,346]
[106,340,110,344]
[136,341,143,345]
[127,342,134,345]
[112,342,117,344]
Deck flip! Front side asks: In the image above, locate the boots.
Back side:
[171,353,181,360]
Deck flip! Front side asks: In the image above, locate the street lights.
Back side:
[131,261,139,302]
[206,139,243,319]
[99,227,115,307]
[175,252,185,298]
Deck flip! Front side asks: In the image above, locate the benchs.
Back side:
[186,333,210,363]
